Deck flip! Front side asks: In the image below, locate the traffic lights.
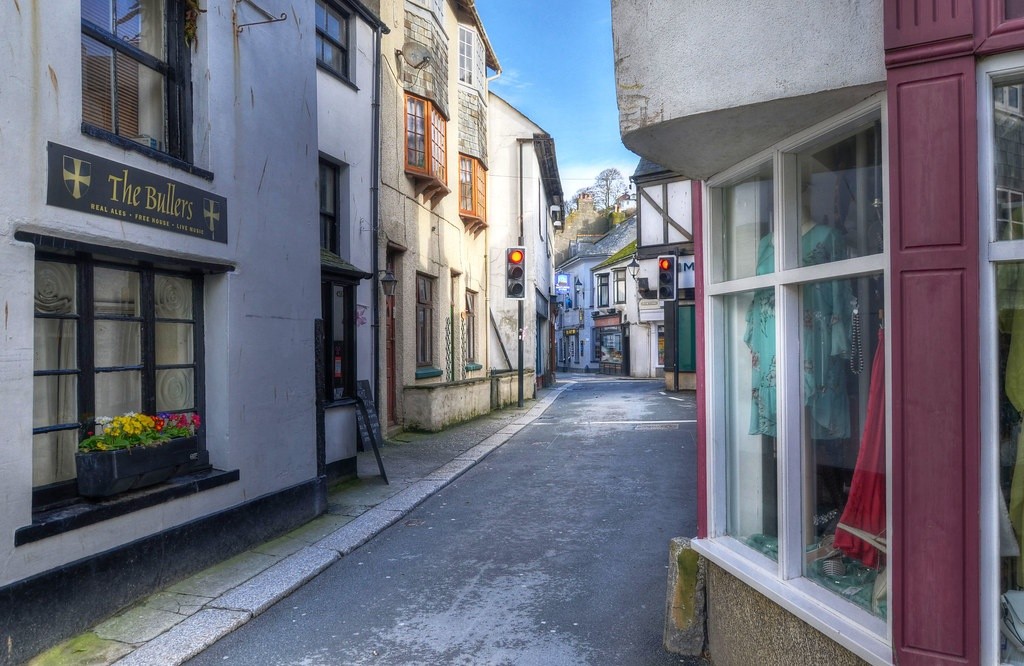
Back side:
[504,246,527,301]
[657,255,679,302]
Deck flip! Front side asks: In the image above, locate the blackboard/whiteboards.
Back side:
[355,379,382,452]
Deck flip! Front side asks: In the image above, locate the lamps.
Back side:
[378,269,398,297]
[627,253,641,276]
[578,307,584,324]
[575,278,582,294]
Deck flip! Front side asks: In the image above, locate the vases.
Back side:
[74,435,201,501]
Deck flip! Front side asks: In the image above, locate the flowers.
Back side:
[77,410,200,454]
